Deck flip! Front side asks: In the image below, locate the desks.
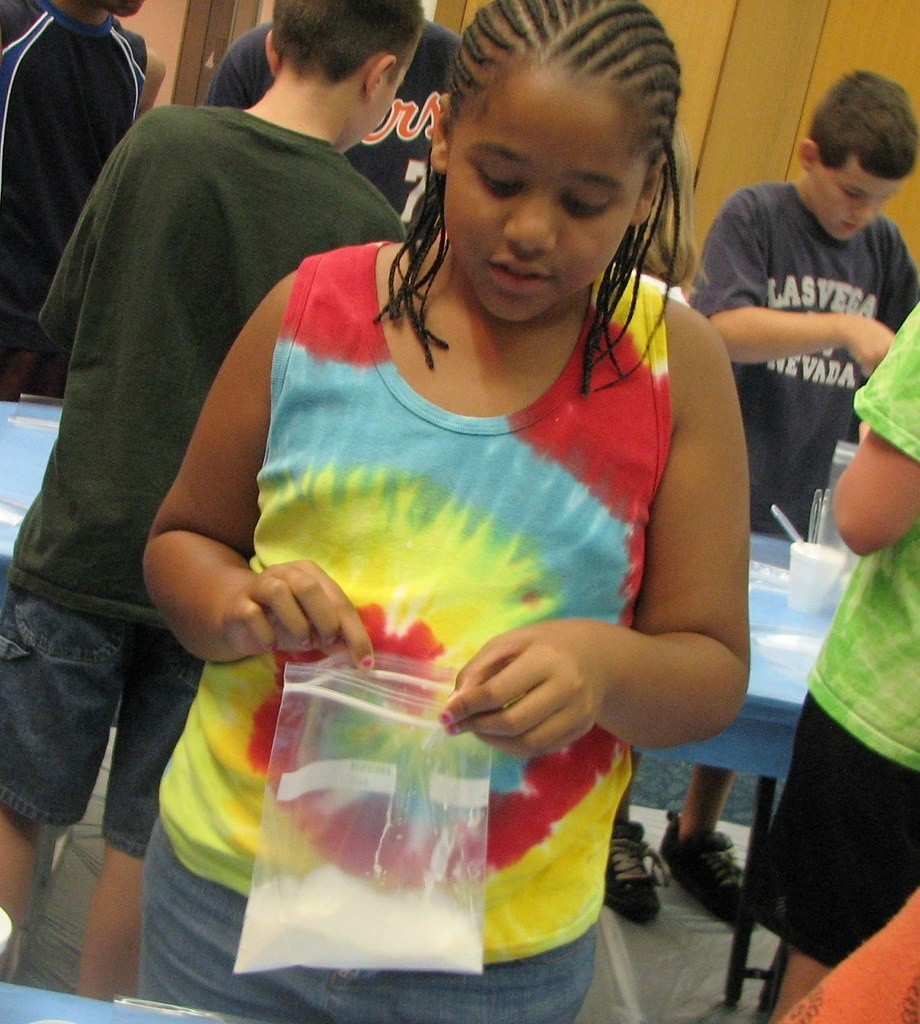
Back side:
[0,402,920,1024]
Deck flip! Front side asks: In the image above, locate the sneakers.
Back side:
[603,819,671,924]
[660,808,748,927]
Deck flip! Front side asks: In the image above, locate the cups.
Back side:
[789,543,844,614]
[817,440,858,551]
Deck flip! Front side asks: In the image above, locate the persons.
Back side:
[605,70,920,1024]
[0,0,752,1024]
